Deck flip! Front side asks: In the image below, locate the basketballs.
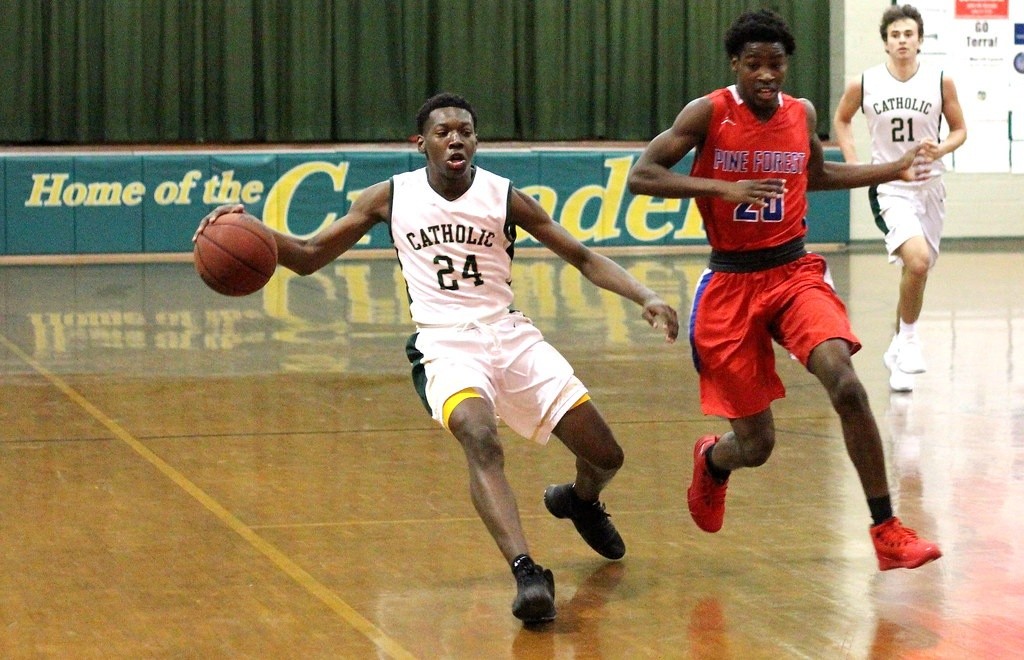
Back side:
[193,214,279,297]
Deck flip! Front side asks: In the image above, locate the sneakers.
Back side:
[687,434,729,533]
[897,336,929,373]
[511,563,559,630]
[869,515,943,571]
[544,482,626,560]
[883,350,915,393]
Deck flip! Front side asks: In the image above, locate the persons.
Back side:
[193,95,678,621]
[886,393,938,533]
[834,3,966,392]
[627,7,942,571]
